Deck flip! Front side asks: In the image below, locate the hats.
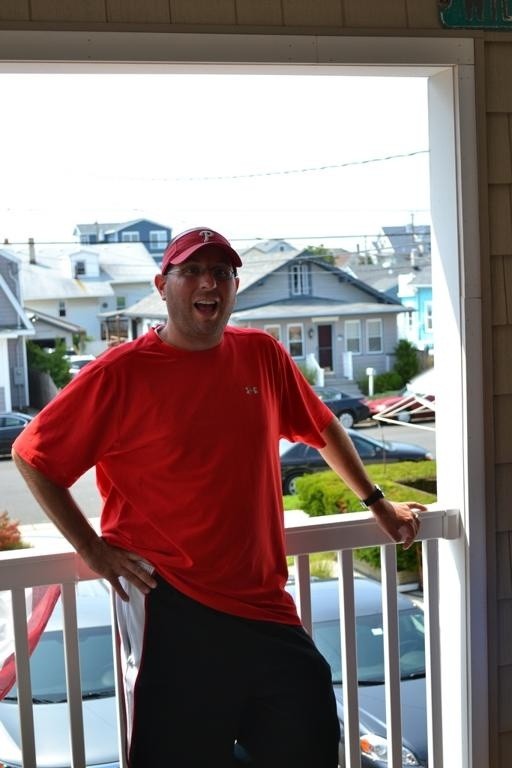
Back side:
[162,227,242,275]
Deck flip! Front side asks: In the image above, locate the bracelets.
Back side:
[361,484,385,508]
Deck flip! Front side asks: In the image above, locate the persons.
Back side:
[10,227,430,767]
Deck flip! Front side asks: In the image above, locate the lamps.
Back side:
[308,327,314,339]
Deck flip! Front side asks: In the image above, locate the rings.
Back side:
[414,512,419,520]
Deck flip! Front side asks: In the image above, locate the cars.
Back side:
[0,411,33,457]
[279,427,433,495]
[282,575,428,766]
[0,543,121,768]
[310,385,369,428]
[365,385,435,425]
[61,354,96,377]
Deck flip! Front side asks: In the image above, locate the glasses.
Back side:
[168,260,235,282]
